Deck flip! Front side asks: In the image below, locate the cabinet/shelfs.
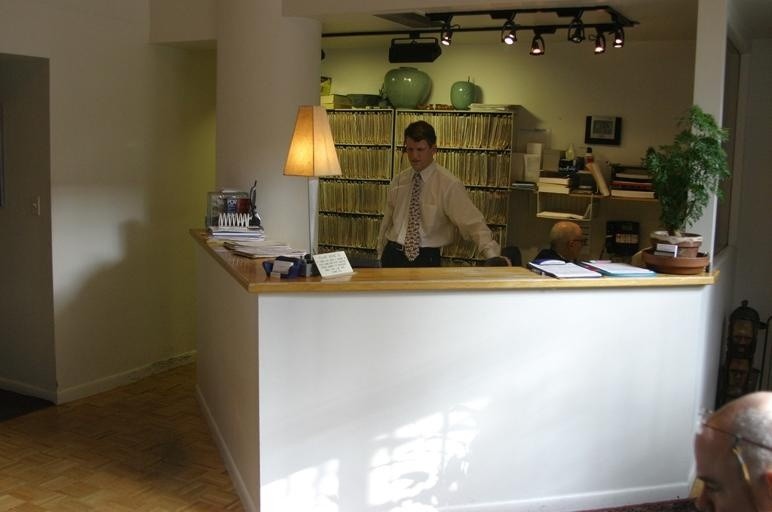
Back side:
[316,107,393,265]
[393,109,514,268]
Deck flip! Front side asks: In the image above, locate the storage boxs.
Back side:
[320,94,352,110]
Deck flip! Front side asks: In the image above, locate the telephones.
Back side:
[606,221,640,255]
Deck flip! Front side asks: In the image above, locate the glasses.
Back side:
[568,237,588,246]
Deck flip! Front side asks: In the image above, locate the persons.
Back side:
[689,386,771,511]
[532,221,584,262]
[377,123,512,268]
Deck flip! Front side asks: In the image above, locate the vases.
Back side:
[383,66,434,109]
[450,79,478,111]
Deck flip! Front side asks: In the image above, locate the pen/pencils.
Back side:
[527,265,545,276]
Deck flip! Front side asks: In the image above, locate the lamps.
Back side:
[423,5,640,58]
[282,101,344,277]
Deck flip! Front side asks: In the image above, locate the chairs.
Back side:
[480,254,512,267]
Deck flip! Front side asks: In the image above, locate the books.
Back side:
[319,110,506,267]
[530,260,656,281]
[609,164,658,198]
[537,174,573,196]
[228,237,310,259]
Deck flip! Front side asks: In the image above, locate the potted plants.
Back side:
[643,103,730,276]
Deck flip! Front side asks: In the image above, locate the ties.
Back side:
[405,167,426,263]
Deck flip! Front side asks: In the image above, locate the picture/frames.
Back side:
[584,113,622,146]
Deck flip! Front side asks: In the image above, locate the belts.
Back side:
[386,242,440,257]
